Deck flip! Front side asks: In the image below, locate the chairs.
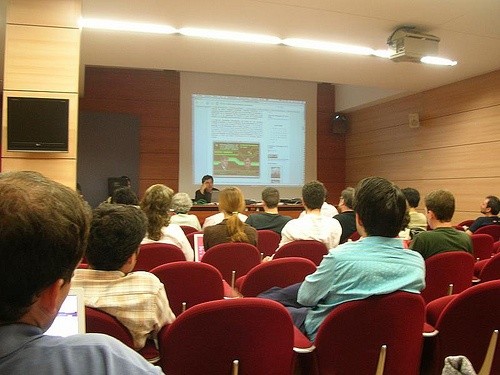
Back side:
[74,219,500,375]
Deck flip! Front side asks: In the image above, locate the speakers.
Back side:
[409,112,419,128]
[331,119,346,134]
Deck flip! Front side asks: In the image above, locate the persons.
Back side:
[0,170,167,375]
[70,174,499,375]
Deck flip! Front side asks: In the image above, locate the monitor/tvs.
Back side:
[7,96,69,150]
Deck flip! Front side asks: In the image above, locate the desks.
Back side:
[168,204,306,219]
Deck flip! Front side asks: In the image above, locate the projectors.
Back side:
[389,36,439,64]
